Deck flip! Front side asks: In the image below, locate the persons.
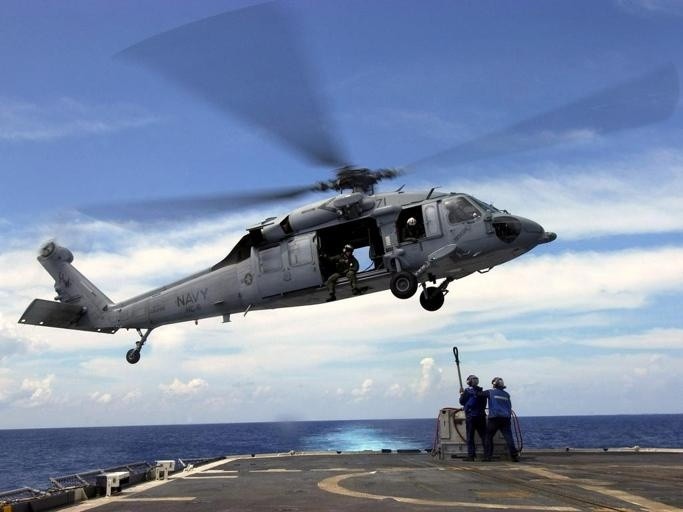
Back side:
[318,243,361,302]
[457,374,489,462]
[398,214,425,244]
[474,376,519,463]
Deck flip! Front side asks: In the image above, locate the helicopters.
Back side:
[15,1,683,366]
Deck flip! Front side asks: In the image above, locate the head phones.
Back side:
[342,248,346,253]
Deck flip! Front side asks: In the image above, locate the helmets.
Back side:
[342,244,352,253]
[406,217,416,226]
[466,375,478,385]
[491,377,503,387]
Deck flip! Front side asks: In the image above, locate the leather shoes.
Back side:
[511,458,518,462]
[351,290,357,295]
[325,296,336,302]
[480,457,490,462]
[462,457,474,462]
